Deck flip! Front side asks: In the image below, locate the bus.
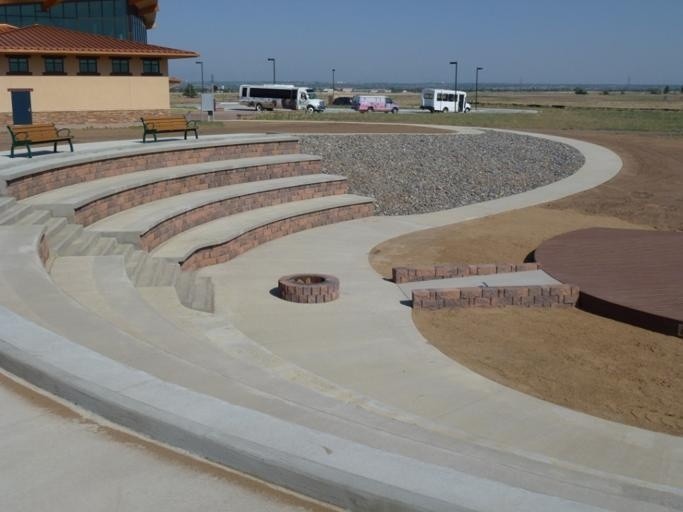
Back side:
[238,84,325,113]
[420,88,471,113]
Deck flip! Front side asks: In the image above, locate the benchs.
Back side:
[140,114,202,143]
[5,122,74,158]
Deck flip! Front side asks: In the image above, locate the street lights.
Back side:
[195,61,203,93]
[449,61,457,92]
[475,67,483,110]
[267,57,275,85]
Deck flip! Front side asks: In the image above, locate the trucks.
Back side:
[329,68,335,99]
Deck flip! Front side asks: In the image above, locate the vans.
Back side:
[351,94,400,114]
